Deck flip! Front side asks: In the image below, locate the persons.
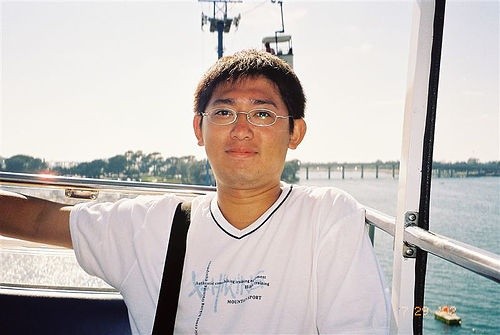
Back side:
[0,49,398,335]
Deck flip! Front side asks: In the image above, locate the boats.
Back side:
[434,304,461,325]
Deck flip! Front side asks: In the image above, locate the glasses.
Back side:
[197,108,292,127]
